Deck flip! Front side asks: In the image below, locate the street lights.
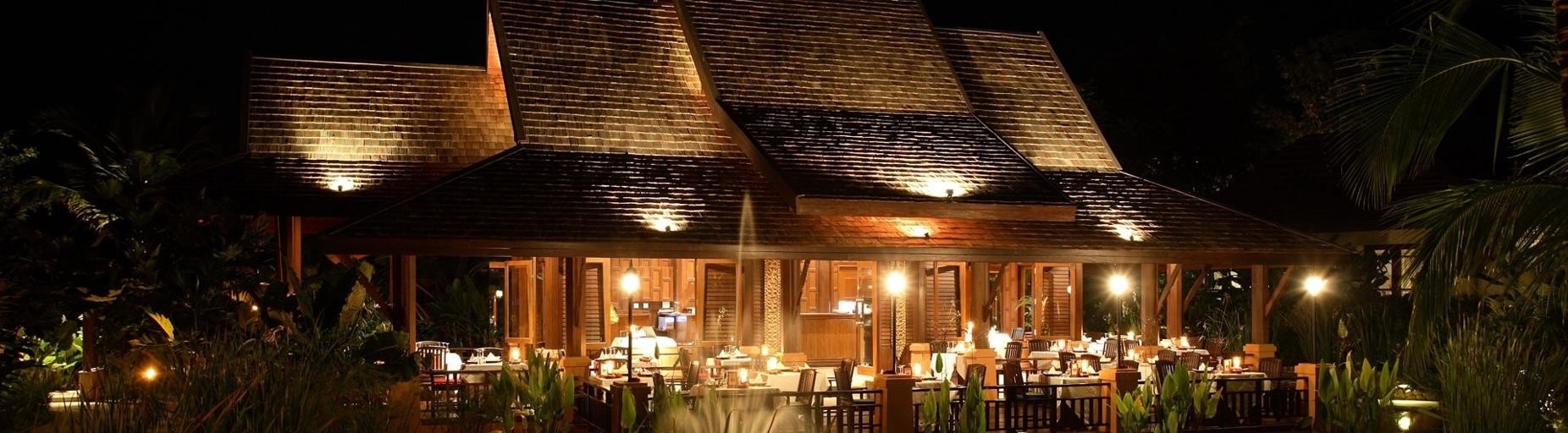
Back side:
[1304,275,1327,362]
[621,260,641,382]
[1107,274,1132,370]
[883,272,906,374]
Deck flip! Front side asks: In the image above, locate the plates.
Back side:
[462,361,479,364]
[1043,371,1064,376]
[486,359,502,363]
[1193,367,1214,373]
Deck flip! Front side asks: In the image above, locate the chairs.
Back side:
[413,338,1283,433]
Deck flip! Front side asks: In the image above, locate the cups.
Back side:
[478,349,484,365]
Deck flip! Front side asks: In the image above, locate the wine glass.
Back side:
[1064,360,1072,377]
[946,340,955,354]
[1216,357,1224,373]
[1050,340,1058,352]
[441,342,450,354]
[724,345,737,361]
